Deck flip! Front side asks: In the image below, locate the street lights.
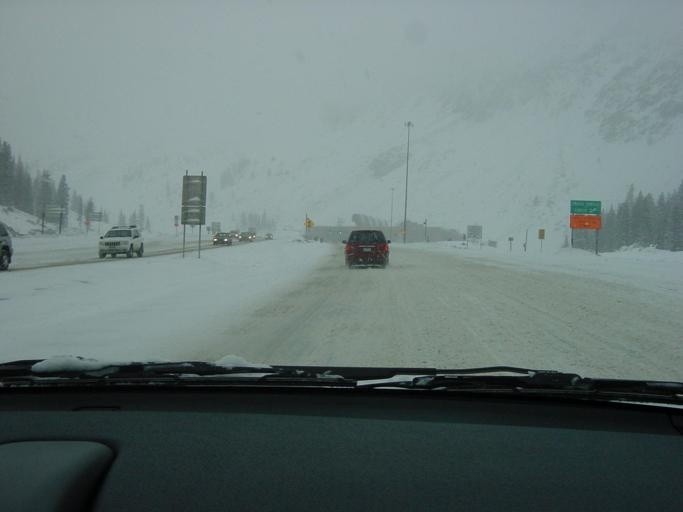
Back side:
[389,187,395,227]
[402,120,416,243]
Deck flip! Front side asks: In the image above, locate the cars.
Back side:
[210,228,273,245]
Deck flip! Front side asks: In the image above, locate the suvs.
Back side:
[98,224,144,258]
[0,222,12,269]
[342,229,390,269]
